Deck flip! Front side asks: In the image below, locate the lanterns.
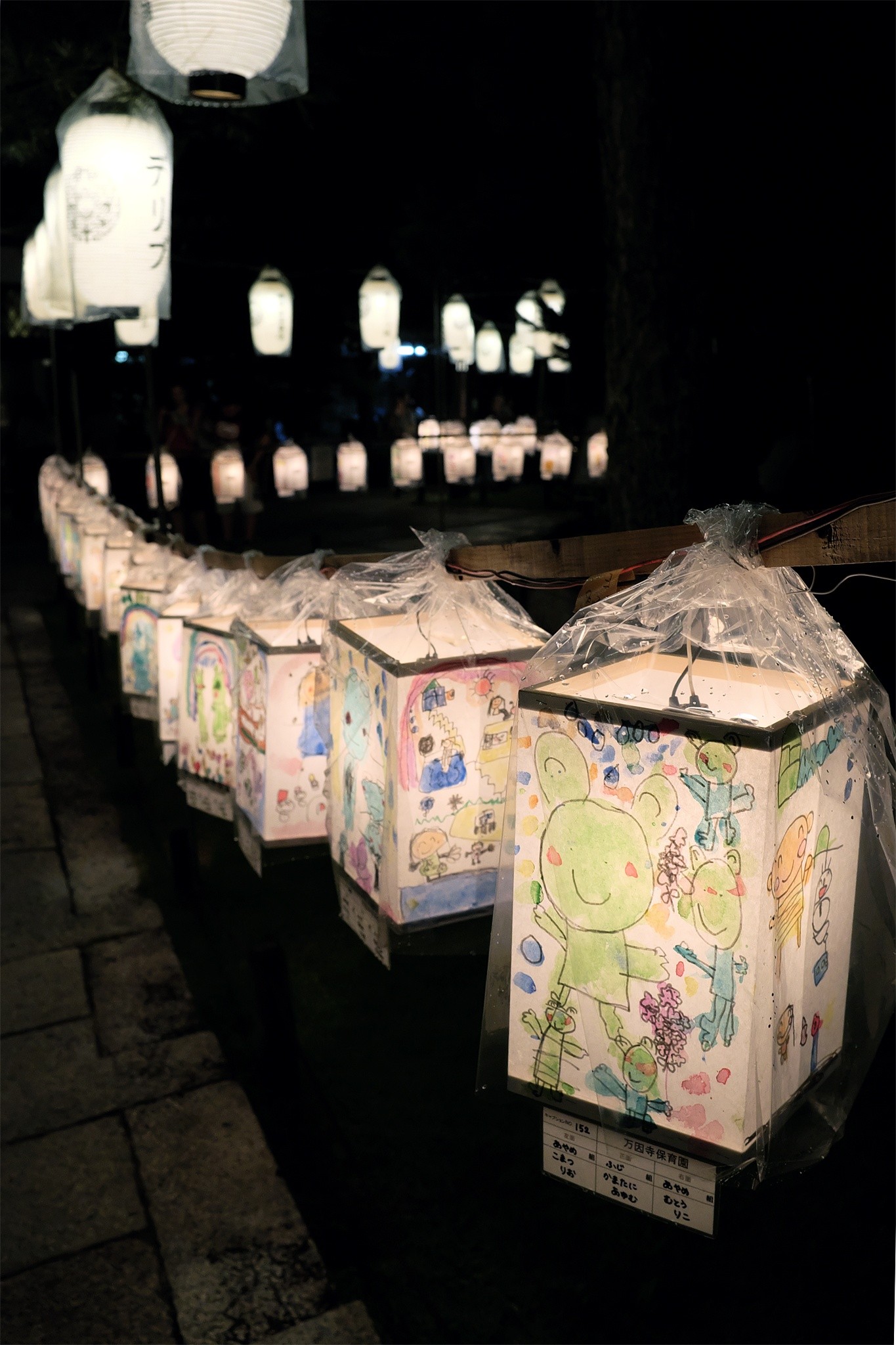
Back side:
[19,0,874,1167]
